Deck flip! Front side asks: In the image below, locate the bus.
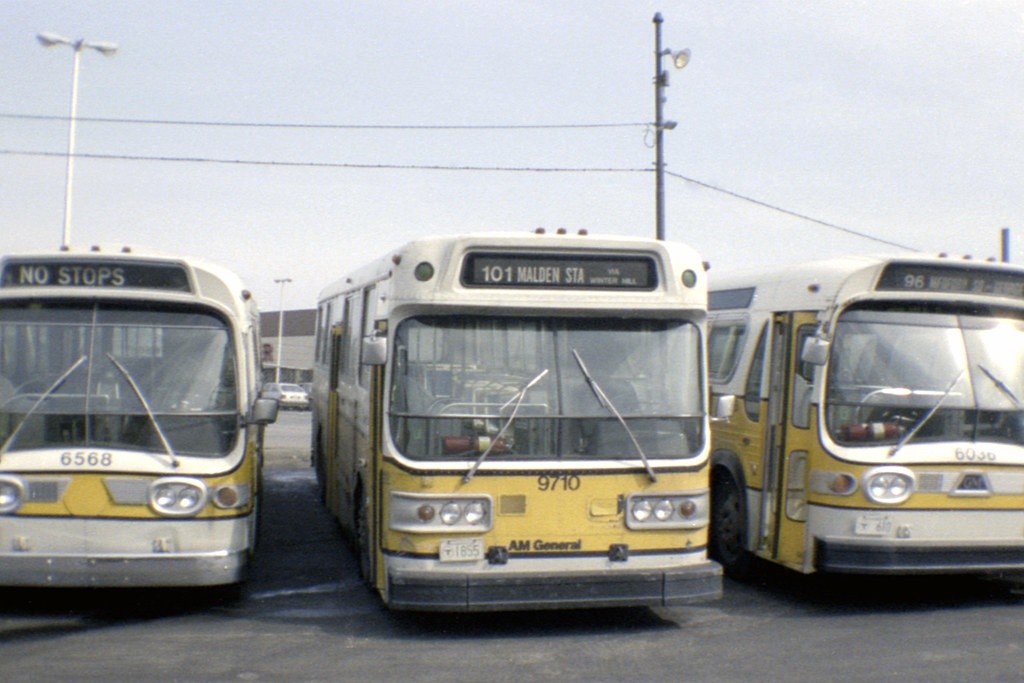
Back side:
[703,260,1023,577]
[0,253,282,606]
[309,236,723,612]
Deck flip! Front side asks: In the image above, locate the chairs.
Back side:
[452,366,527,448]
[10,379,45,445]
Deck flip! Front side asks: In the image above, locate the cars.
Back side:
[260,382,310,411]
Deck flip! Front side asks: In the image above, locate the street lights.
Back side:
[36,31,118,250]
[651,11,690,239]
[273,277,292,382]
